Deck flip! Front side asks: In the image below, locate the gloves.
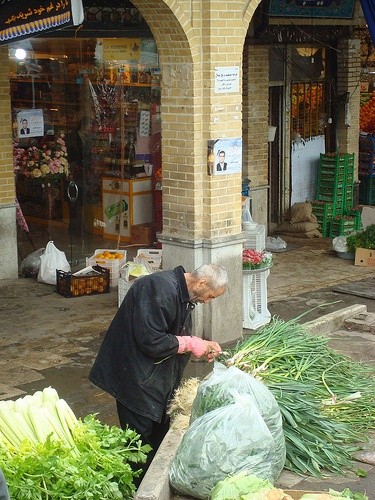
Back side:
[175,335,211,359]
[204,340,222,362]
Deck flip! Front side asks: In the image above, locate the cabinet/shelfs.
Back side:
[9,75,164,242]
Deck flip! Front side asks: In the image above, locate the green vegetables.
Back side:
[0,386,153,500]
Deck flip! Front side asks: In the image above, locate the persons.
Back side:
[20,119,30,134]
[88,263,229,493]
[217,151,227,171]
[292,100,310,130]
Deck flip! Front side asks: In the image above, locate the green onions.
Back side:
[218,299,374,477]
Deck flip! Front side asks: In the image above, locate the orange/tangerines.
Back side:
[93,251,124,260]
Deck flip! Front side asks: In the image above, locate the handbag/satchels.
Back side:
[19,247,46,278]
[36,240,72,285]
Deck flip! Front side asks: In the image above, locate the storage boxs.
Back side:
[134,249,162,270]
[85,249,127,288]
[56,265,111,299]
[241,268,272,330]
[353,246,375,268]
[118,276,137,308]
[241,223,266,254]
[308,150,366,239]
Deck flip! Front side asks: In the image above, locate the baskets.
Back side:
[242,267,272,330]
[85,248,127,280]
[134,248,163,269]
[117,276,139,309]
[56,263,111,297]
[305,151,361,239]
